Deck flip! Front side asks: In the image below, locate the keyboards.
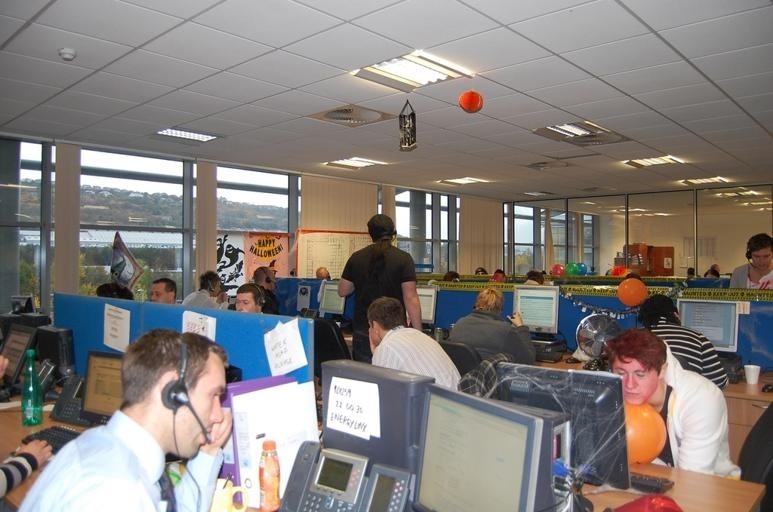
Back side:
[22,426,79,457]
[629,468,675,494]
[535,350,564,364]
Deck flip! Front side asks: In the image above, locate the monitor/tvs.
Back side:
[497,364,631,492]
[678,298,739,358]
[412,385,544,512]
[319,282,346,315]
[406,284,438,326]
[513,284,560,341]
[80,352,126,419]
[11,294,35,315]
[0,325,35,386]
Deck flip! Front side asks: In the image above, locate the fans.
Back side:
[575,314,622,371]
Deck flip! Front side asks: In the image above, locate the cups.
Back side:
[210,480,248,511]
[744,364,761,385]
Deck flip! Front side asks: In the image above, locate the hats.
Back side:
[368,215,396,240]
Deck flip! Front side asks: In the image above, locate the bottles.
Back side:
[21,349,42,427]
[259,440,281,511]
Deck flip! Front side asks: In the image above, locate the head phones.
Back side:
[265,273,272,285]
[744,250,752,258]
[158,337,190,409]
[259,295,267,308]
[208,284,215,294]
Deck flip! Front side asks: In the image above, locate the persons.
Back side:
[368,295,461,391]
[607,326,741,479]
[450,287,535,364]
[17,329,233,512]
[687,264,720,278]
[729,233,773,289]
[0,355,53,503]
[443,267,544,286]
[639,294,729,389]
[95,264,350,387]
[337,214,423,365]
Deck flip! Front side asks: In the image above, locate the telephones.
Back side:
[300,308,318,319]
[279,440,411,512]
[49,376,111,428]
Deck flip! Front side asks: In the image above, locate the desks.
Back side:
[722,368,773,464]
[0,400,86,512]
[581,462,767,511]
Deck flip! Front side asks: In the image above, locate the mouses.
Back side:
[761,383,773,393]
[567,357,577,364]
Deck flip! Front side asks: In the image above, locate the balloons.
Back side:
[616,276,648,307]
[624,402,667,462]
[553,264,587,276]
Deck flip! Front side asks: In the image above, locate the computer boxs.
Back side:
[321,360,436,473]
[515,405,572,512]
[37,324,76,387]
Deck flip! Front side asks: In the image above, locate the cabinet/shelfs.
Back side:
[622,244,674,276]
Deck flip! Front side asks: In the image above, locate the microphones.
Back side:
[188,401,213,444]
[747,261,757,271]
